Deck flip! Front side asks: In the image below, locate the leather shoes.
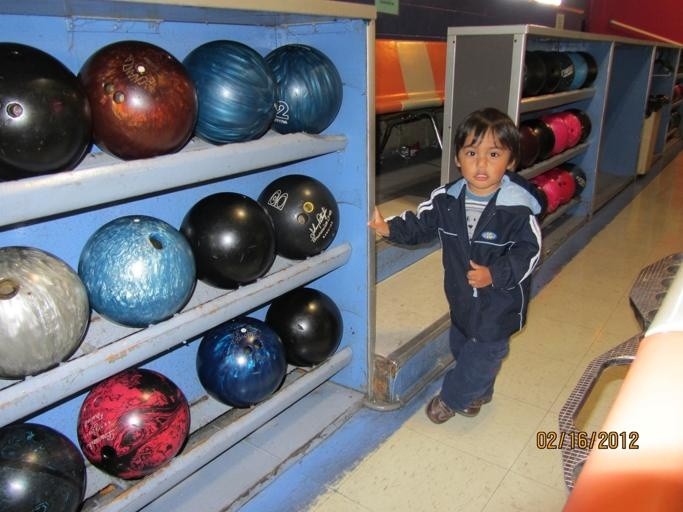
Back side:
[455,393,491,417]
[427,393,456,424]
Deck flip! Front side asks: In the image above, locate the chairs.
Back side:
[372,39,447,161]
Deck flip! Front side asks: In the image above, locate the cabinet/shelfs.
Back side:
[0,1,375,512]
[441,26,683,243]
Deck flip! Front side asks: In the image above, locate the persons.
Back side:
[367,107,542,424]
